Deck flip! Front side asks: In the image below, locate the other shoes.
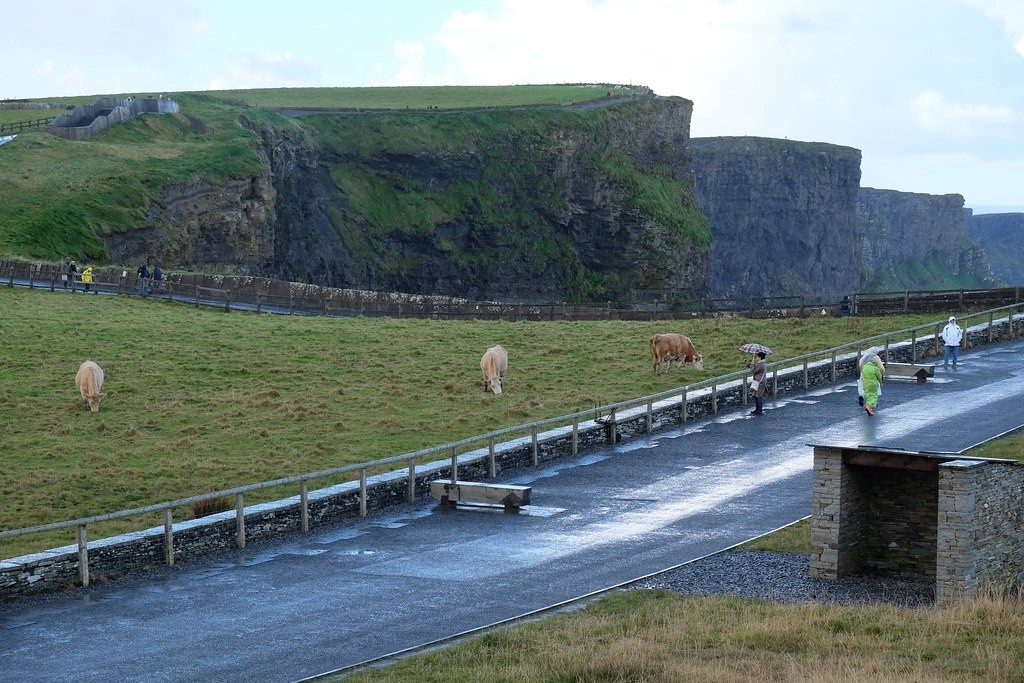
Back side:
[952,364,957,368]
[751,409,763,415]
[865,405,873,416]
[858,396,864,406]
[943,363,948,368]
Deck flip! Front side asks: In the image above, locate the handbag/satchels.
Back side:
[750,380,760,391]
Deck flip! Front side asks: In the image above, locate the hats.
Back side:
[867,356,875,363]
[756,352,766,359]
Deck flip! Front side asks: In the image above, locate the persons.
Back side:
[61,257,78,289]
[942,316,963,371]
[857,346,886,416]
[136,264,163,295]
[81,267,92,295]
[744,352,767,416]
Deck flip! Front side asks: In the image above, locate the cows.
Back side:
[649,332,703,372]
[75,360,107,412]
[480,344,508,394]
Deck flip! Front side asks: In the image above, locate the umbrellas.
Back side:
[739,343,773,366]
